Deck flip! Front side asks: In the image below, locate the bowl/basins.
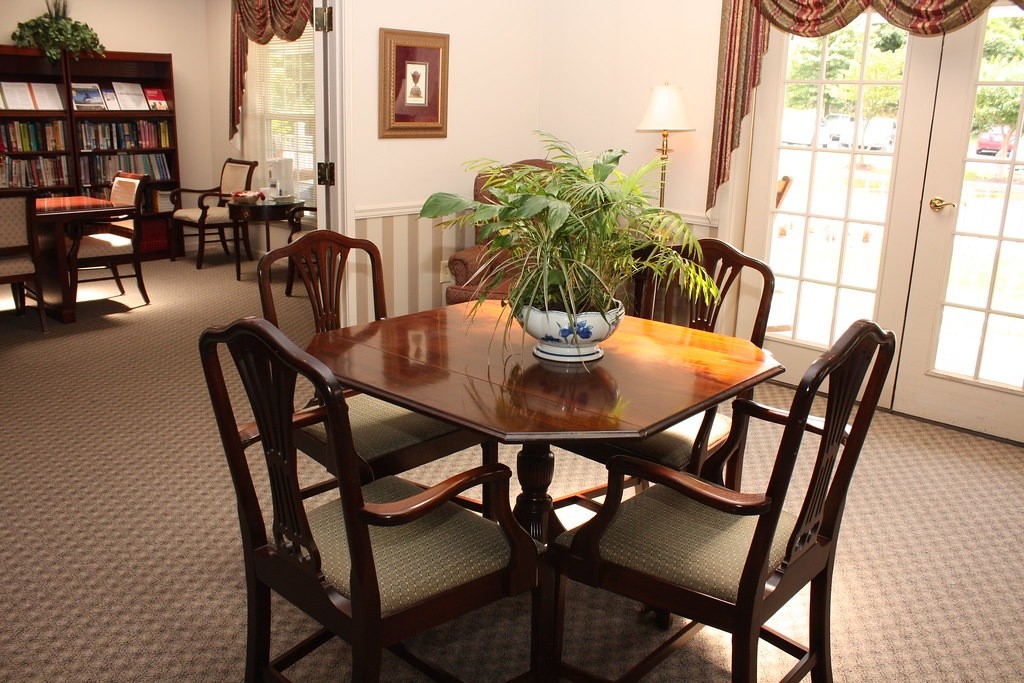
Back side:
[231,191,259,203]
[272,195,297,202]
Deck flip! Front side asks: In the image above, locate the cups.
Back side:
[260,187,269,201]
[266,178,280,200]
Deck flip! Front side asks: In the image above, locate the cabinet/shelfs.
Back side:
[0,44,185,268]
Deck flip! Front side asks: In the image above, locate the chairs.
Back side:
[548,239,774,511]
[285,207,317,296]
[65,171,152,304]
[168,158,258,269]
[549,320,895,683]
[0,187,49,332]
[257,230,498,523]
[775,175,793,209]
[197,316,554,683]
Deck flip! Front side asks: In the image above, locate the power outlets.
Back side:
[440,261,451,284]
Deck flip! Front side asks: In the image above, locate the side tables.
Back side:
[629,241,692,318]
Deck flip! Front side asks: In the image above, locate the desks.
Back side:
[228,199,306,282]
[24,195,138,324]
[293,299,785,546]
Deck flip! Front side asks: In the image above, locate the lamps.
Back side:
[636,82,695,240]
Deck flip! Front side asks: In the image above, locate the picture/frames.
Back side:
[377,27,449,139]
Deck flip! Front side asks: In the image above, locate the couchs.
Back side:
[447,159,559,305]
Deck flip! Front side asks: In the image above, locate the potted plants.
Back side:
[463,354,629,431]
[415,132,723,374]
[11,0,106,65]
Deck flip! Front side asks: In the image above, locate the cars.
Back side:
[818,113,855,146]
[976,122,1018,154]
[779,110,818,147]
[856,116,900,150]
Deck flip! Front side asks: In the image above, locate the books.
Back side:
[0,81,176,213]
